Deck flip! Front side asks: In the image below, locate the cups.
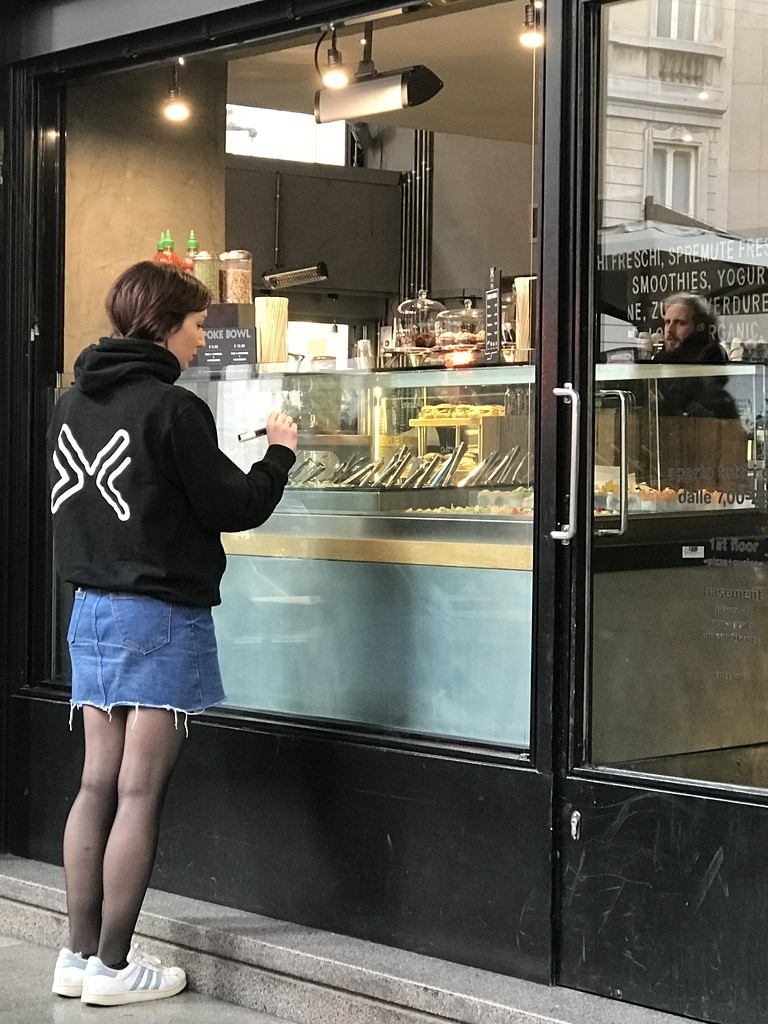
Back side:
[637,329,652,359]
[652,333,665,346]
[357,338,374,369]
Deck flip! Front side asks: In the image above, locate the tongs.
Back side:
[286,440,534,486]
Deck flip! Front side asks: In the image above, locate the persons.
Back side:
[642,289,738,418]
[41,257,300,1011]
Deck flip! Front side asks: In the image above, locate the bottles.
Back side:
[155,228,179,264]
[180,230,201,274]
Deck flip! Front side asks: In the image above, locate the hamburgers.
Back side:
[423,452,443,472]
[432,404,455,418]
[484,405,505,417]
[467,406,492,418]
[443,453,452,460]
[452,404,471,418]
[417,404,433,419]
[457,452,477,470]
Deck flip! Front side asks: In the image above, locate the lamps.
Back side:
[262,261,330,290]
[313,2,444,123]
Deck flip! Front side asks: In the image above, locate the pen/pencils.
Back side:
[238,428,267,443]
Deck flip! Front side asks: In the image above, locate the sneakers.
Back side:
[51,947,92,998]
[80,947,188,1006]
